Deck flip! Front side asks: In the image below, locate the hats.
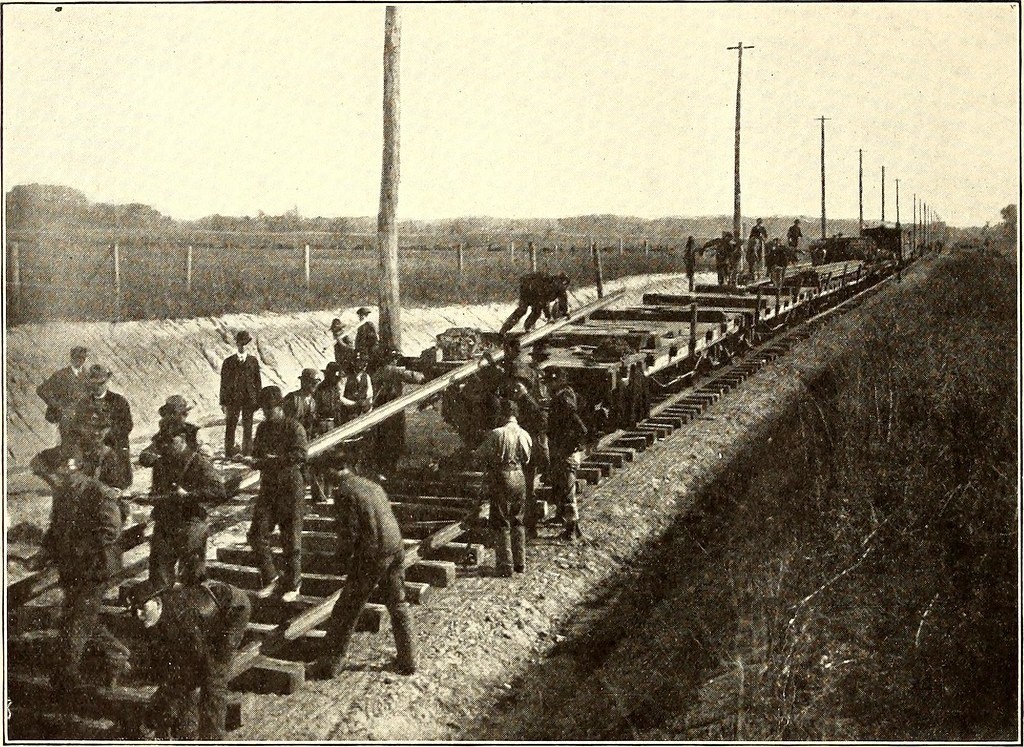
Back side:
[234,330,252,345]
[298,368,321,382]
[118,582,170,615]
[321,362,345,378]
[82,364,113,384]
[257,386,284,405]
[77,407,110,428]
[538,366,567,379]
[356,308,371,316]
[328,318,346,331]
[159,395,193,416]
[152,417,186,442]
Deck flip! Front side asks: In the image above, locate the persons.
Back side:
[4,218,847,740]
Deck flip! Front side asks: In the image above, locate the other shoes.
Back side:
[514,564,525,573]
[319,666,338,679]
[255,579,280,600]
[557,529,576,541]
[399,663,416,675]
[491,565,514,578]
[282,586,300,602]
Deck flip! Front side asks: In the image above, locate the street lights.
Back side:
[725,41,754,239]
[813,116,832,239]
